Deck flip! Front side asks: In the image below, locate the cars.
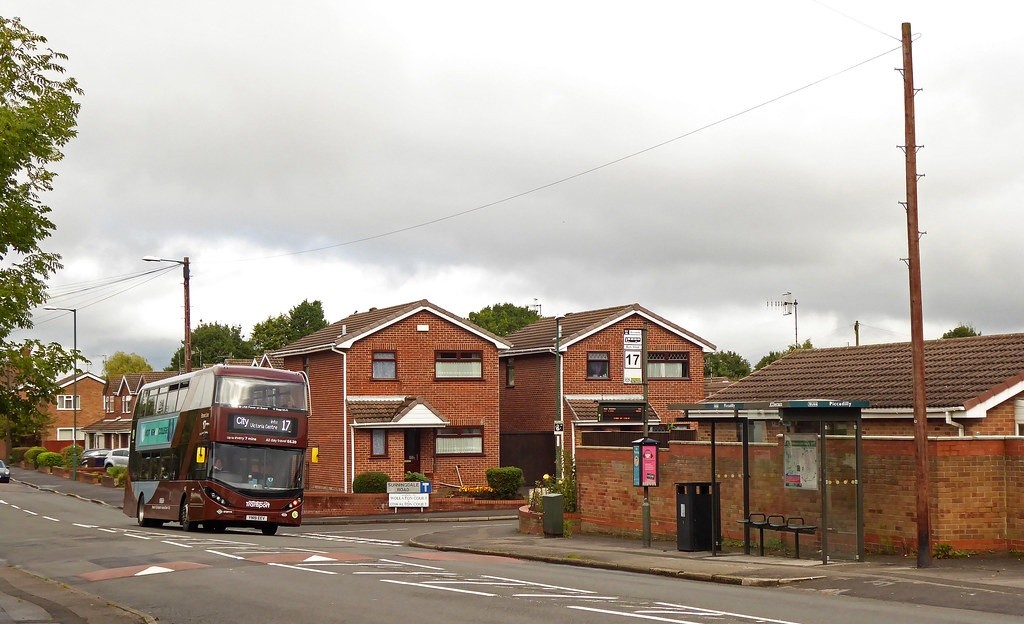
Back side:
[0,460,11,483]
[79,448,129,470]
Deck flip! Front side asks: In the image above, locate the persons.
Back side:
[212,459,224,473]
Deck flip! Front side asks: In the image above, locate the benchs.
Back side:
[736,512,818,558]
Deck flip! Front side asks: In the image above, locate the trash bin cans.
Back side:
[674,482,722,551]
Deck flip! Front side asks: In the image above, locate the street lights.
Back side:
[141,255,192,373]
[554,314,567,493]
[43,307,77,480]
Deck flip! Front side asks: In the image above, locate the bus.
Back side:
[123,363,320,535]
